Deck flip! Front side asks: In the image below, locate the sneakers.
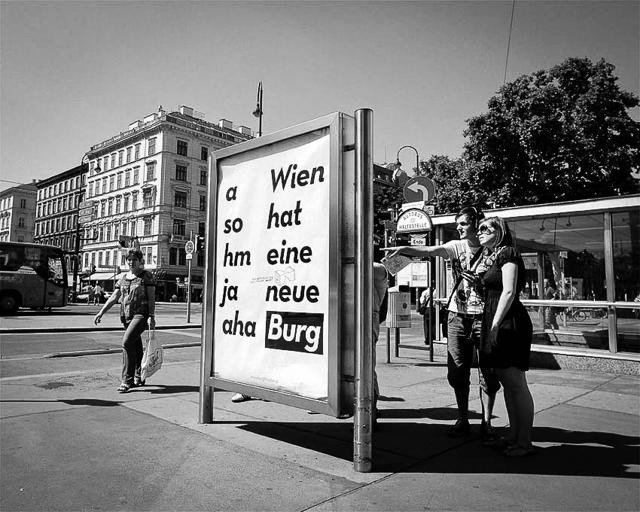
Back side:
[478,419,497,446]
[442,417,469,434]
[232,392,251,402]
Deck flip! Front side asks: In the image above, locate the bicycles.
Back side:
[559,308,589,323]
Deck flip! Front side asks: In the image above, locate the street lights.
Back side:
[395,144,424,310]
[253,80,266,136]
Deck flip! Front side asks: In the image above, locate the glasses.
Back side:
[480,224,497,234]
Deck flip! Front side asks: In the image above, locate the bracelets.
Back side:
[148,314,155,318]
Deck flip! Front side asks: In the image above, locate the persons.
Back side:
[473,214,538,459]
[419,278,437,346]
[542,277,560,330]
[375,205,501,447]
[372,260,390,405]
[92,283,102,306]
[94,248,158,393]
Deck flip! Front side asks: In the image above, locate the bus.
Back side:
[1,240,70,313]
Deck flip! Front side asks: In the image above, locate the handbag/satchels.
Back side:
[140,337,164,382]
[419,305,427,315]
[441,306,450,338]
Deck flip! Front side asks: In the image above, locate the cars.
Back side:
[69,287,121,304]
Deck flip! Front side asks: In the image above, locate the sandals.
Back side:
[116,376,145,391]
[499,431,534,458]
